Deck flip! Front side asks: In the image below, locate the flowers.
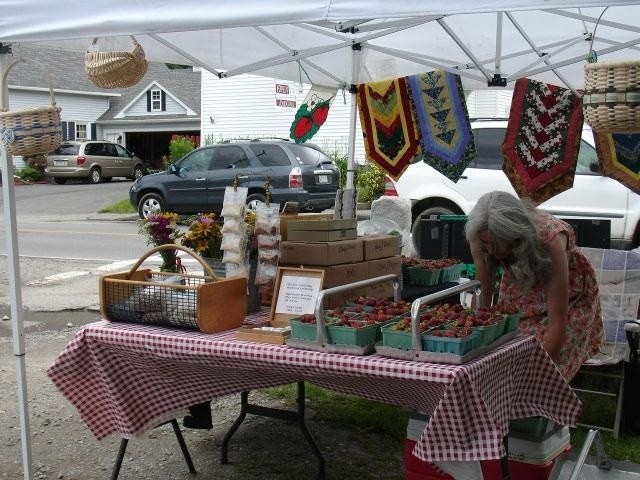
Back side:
[181,210,260,259]
[135,209,185,274]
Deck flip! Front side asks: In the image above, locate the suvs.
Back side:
[45,140,144,182]
[129,138,341,220]
[385,116,639,256]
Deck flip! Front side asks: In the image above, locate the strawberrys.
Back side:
[399,256,458,272]
[302,296,515,339]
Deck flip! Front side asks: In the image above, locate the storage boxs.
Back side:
[295,255,404,309]
[280,239,364,265]
[404,416,573,480]
[234,266,325,345]
[285,219,358,241]
[359,233,398,261]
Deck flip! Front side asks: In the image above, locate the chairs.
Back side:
[544,246,639,441]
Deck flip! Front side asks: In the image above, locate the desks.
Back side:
[47,301,582,479]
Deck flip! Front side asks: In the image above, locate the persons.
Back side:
[465,192,607,385]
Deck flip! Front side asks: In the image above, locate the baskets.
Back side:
[84,34,149,89]
[1,56,62,157]
[98,243,248,335]
[582,63,640,134]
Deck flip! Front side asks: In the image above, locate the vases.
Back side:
[158,262,186,295]
[203,256,261,313]
